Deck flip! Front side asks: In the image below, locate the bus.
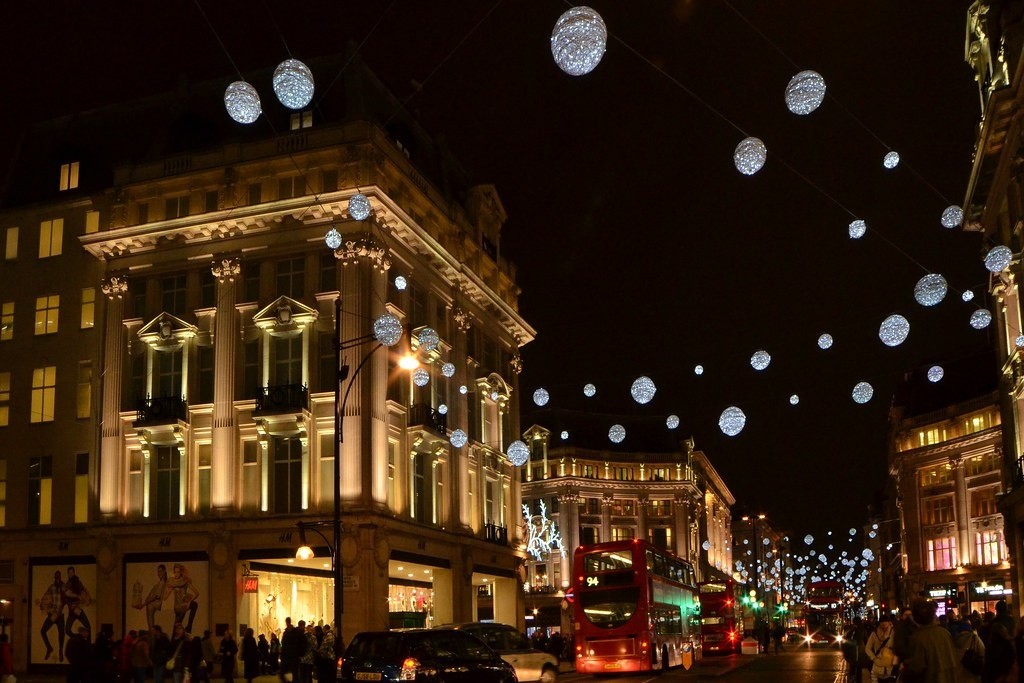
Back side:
[803,580,846,648]
[573,538,759,673]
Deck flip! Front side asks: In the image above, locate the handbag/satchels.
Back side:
[865,660,874,671]
[961,635,984,676]
[166,659,176,671]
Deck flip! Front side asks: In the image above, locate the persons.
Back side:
[530,630,574,669]
[38,562,201,663]
[753,595,1024,683]
[0,618,339,683]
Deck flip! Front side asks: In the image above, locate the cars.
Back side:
[338,622,561,683]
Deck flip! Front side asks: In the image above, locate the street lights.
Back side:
[772,545,787,634]
[291,296,420,661]
[741,513,766,603]
